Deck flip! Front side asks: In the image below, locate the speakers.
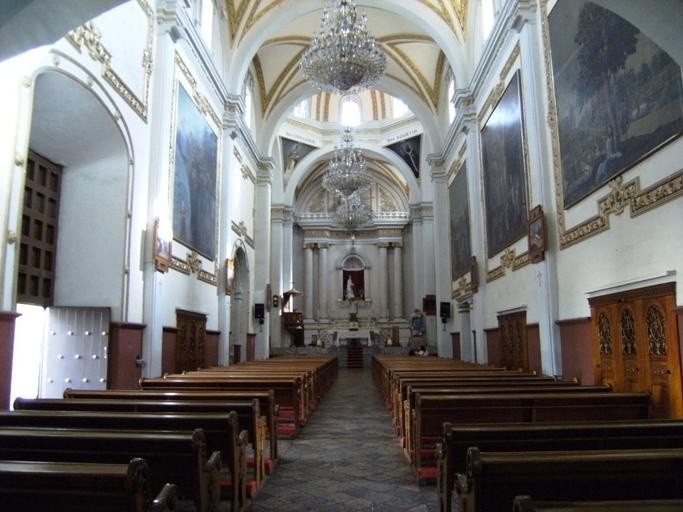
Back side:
[440,302,451,318]
[255,304,264,319]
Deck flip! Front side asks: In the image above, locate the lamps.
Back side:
[322,95,375,233]
[296,1,389,95]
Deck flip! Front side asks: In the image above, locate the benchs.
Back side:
[370,351,682,512]
[0,351,339,512]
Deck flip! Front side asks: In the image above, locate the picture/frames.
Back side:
[226,257,237,296]
[528,205,547,263]
[152,216,174,275]
[470,256,479,292]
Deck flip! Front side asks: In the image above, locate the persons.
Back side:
[408,309,429,356]
[345,275,354,299]
